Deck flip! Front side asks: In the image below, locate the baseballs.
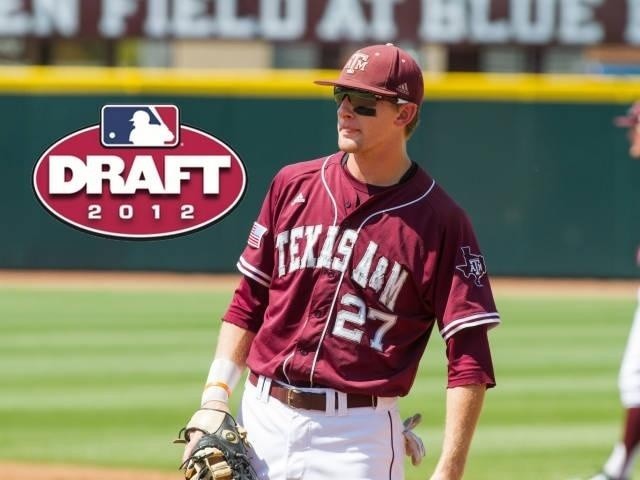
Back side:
[109,132,116,138]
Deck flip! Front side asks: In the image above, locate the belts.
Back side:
[249,369,378,411]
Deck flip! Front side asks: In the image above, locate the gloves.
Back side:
[402,414,425,466]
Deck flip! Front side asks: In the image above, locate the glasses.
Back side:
[334,86,412,118]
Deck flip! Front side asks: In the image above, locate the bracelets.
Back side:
[201,382,236,399]
[200,357,240,407]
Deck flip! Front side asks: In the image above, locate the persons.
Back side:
[587,92,640,479]
[177,41,503,480]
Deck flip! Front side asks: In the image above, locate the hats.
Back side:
[313,44,424,106]
[612,98,640,128]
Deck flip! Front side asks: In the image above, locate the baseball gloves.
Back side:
[174,408,258,480]
